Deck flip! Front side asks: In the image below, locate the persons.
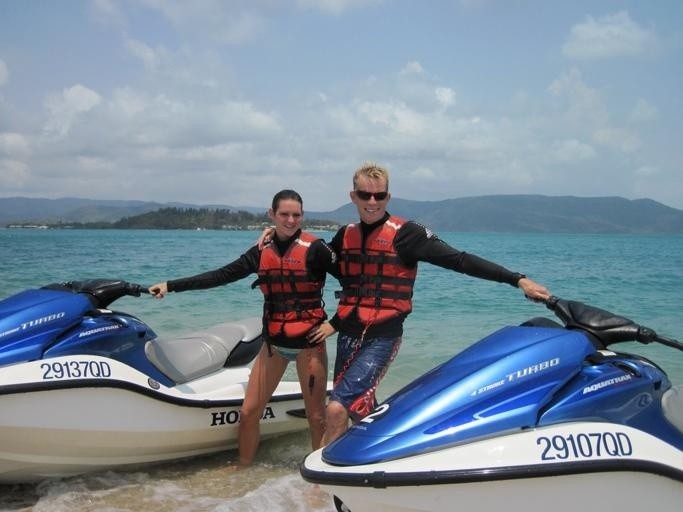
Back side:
[147,189,340,475]
[254,159,551,449]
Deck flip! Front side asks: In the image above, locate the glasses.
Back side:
[354,190,389,202]
